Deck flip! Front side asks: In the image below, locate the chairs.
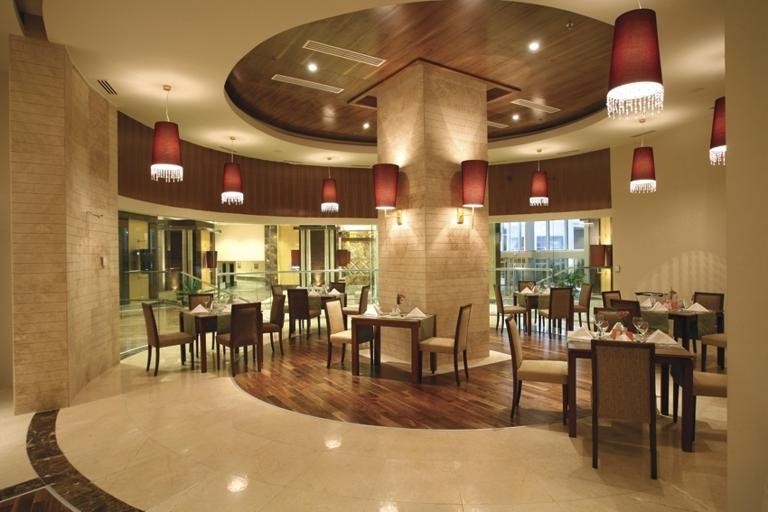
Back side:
[419,304,476,391]
[141,280,372,382]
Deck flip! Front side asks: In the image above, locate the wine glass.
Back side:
[632,317,643,337]
[638,321,649,342]
[202,296,210,309]
[543,280,548,294]
[528,281,534,293]
[599,321,609,339]
[535,281,541,294]
[594,315,604,336]
[373,298,383,317]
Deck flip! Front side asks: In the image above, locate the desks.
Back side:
[347,308,438,388]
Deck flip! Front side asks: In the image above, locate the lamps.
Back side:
[370,162,404,232]
[148,85,186,183]
[628,117,657,196]
[705,97,727,168]
[605,0,667,120]
[220,136,245,207]
[457,160,489,226]
[291,250,300,269]
[320,157,338,214]
[528,146,550,208]
[335,249,347,270]
[590,245,607,275]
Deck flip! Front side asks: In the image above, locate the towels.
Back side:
[406,307,426,319]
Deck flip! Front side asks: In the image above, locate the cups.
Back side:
[550,282,556,288]
[659,294,693,312]
[308,282,329,296]
